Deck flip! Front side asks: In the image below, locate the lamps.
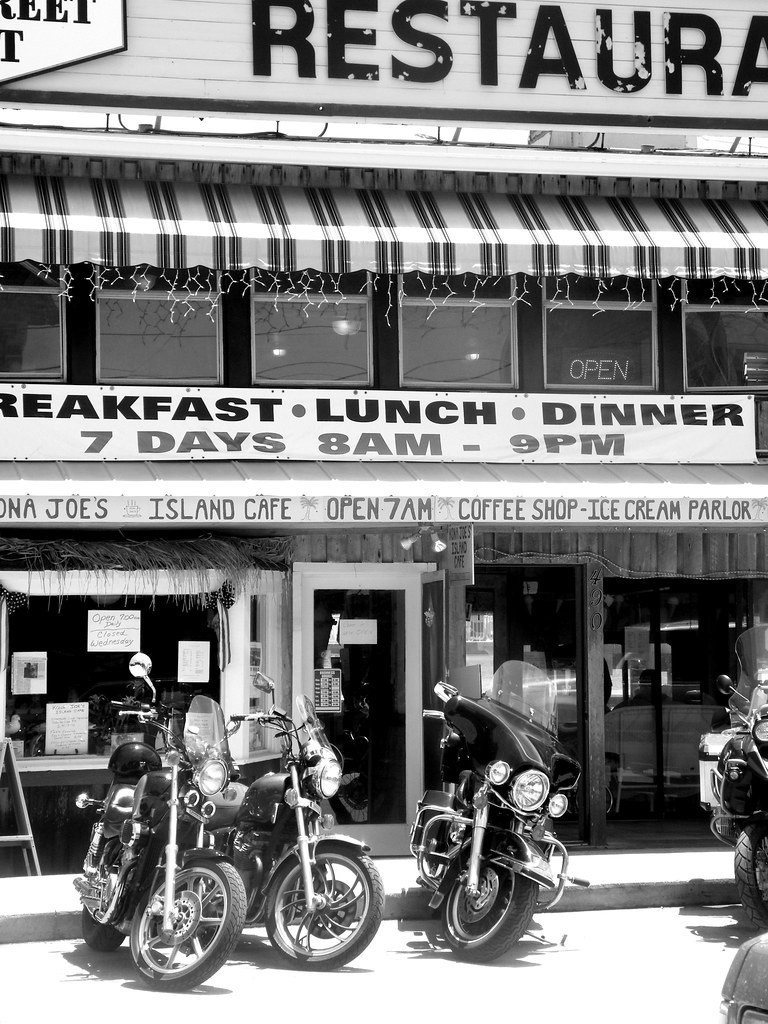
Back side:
[403,523,446,554]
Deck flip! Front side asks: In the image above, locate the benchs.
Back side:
[604,704,725,812]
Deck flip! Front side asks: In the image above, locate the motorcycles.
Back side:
[700,621,768,933]
[410,660,589,962]
[73,652,387,993]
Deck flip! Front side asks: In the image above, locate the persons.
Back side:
[612,669,673,711]
[560,639,612,715]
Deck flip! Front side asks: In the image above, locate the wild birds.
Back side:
[6,714,21,736]
